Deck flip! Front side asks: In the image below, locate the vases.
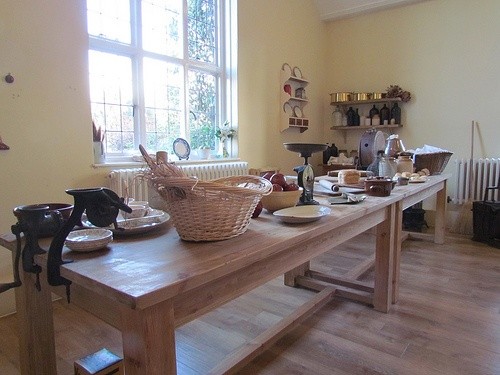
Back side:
[223,139,229,159]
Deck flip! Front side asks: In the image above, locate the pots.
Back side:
[329,92,382,102]
[364,176,393,196]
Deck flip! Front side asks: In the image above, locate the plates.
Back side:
[281,62,303,117]
[272,204,332,224]
[81,207,170,236]
[65,228,114,252]
[173,138,190,158]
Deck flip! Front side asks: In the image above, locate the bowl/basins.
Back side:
[13,202,74,238]
[260,186,303,212]
[120,199,149,220]
[415,152,453,174]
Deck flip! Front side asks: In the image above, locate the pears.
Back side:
[262,168,297,192]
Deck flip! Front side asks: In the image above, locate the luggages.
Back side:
[473,185,500,243]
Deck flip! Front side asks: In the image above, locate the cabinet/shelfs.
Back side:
[280,62,310,135]
[330,97,404,144]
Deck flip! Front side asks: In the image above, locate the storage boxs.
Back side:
[74,347,125,375]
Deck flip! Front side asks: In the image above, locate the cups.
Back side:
[156,151,168,165]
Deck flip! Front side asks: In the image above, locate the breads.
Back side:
[337,169,359,185]
[393,168,430,182]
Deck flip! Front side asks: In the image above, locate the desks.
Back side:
[296,172,452,304]
[0,193,405,375]
[316,163,355,175]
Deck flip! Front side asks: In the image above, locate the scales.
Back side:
[284,142,327,206]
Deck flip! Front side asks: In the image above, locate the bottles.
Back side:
[397,156,413,174]
[323,143,358,165]
[360,143,373,165]
[379,157,398,179]
[331,103,401,126]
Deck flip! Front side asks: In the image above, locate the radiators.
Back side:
[447,157,500,206]
[104,161,248,204]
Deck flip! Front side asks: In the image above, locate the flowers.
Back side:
[214,120,239,144]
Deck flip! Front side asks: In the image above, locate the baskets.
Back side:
[151,173,273,241]
